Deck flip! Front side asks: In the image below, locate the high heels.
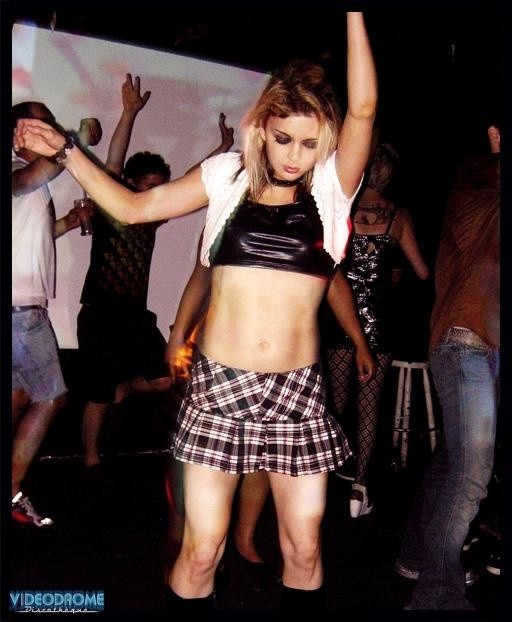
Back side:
[349,483,374,519]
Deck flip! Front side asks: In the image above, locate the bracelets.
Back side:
[47,134,75,165]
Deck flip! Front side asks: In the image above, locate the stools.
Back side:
[390,358,439,470]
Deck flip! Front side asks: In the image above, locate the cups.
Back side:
[74,198,95,237]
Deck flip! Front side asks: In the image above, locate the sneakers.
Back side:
[12,491,55,528]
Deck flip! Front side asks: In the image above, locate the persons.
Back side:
[10,100,104,534]
[71,69,232,482]
[163,225,273,578]
[393,121,500,612]
[13,11,385,621]
[318,137,433,523]
